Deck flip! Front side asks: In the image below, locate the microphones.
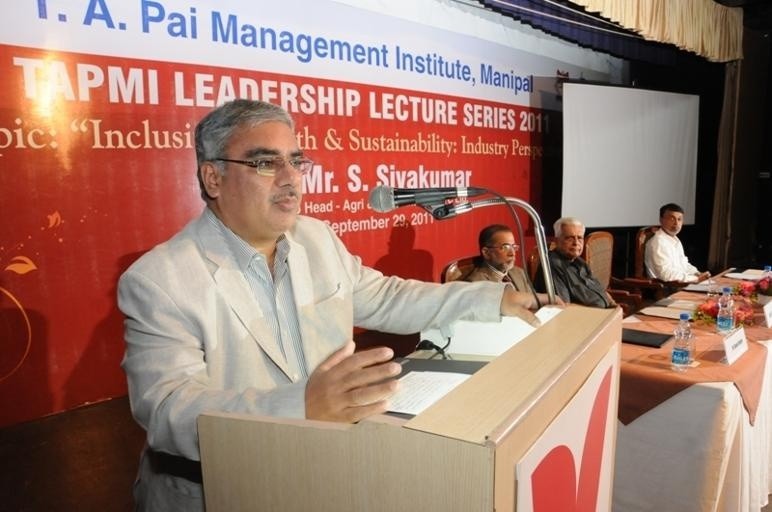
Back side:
[369,185,488,213]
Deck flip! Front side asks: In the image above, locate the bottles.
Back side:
[762,265,772,281]
[673,313,698,373]
[717,287,735,335]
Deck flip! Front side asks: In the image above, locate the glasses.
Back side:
[488,244,520,251]
[209,157,313,176]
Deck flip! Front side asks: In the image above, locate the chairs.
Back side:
[441,225,690,319]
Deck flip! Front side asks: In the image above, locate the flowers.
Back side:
[690,275,770,330]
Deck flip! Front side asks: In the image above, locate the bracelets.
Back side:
[609,300,615,304]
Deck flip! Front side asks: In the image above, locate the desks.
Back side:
[617,265,772,512]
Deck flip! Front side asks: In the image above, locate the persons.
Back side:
[533,217,626,318]
[115,97,567,512]
[463,223,533,292]
[644,203,712,300]
